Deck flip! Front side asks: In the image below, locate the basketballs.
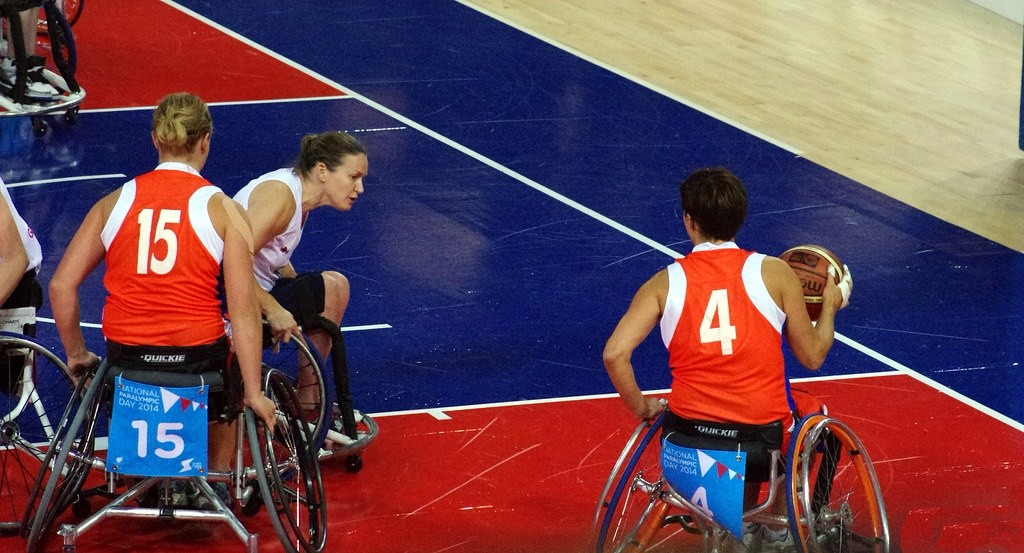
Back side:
[777,244,844,320]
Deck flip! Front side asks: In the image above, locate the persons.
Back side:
[0,0,59,102]
[48,94,276,510]
[604,168,852,553]
[0,179,42,404]
[217,129,368,458]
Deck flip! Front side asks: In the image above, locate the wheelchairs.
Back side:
[0,1,87,138]
[263,315,378,484]
[588,398,891,553]
[21,354,326,553]
[0,307,95,538]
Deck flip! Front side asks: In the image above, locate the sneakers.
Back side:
[162,475,234,509]
[1,54,62,102]
[733,522,800,553]
[296,405,363,443]
